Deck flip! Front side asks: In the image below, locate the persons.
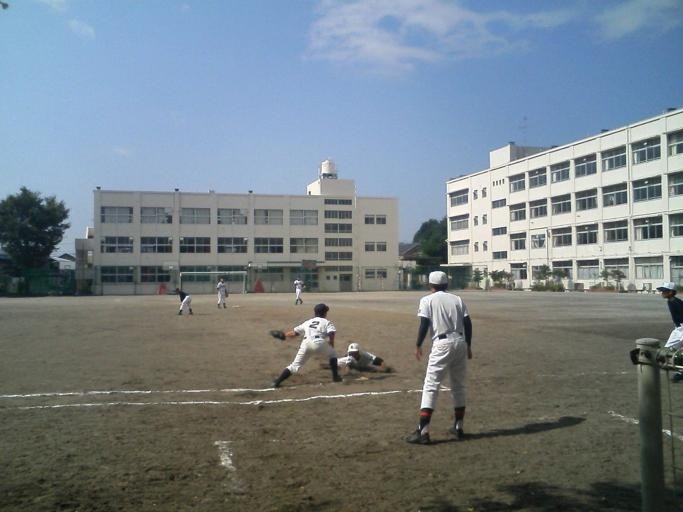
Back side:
[268,303,341,388]
[215,277,228,308]
[654,281,683,382]
[400,270,474,445]
[334,342,384,375]
[292,274,305,305]
[175,287,193,315]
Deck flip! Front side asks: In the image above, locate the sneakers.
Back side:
[332,375,342,381]
[449,426,464,438]
[271,380,279,386]
[407,431,429,443]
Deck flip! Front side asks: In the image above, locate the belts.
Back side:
[303,336,319,339]
[439,332,463,339]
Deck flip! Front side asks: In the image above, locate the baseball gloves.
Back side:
[269,330,285,340]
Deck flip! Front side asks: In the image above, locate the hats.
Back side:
[655,282,677,291]
[428,271,448,285]
[346,343,359,352]
[314,303,329,313]
